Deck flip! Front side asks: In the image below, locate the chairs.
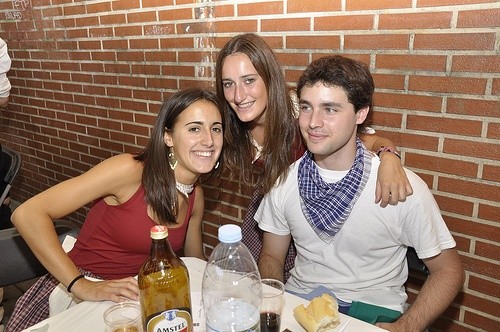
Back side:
[0,221,79,332]
[0,145,21,208]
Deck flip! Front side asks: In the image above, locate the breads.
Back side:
[293,293,340,332]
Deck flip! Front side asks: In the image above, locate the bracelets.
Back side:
[67,275,85,292]
[377,146,401,158]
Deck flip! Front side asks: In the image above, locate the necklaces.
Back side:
[176,183,194,198]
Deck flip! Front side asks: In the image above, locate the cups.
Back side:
[259,278,285,332]
[103,303,143,332]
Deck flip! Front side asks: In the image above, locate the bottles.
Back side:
[137,225,194,332]
[201,224,263,331]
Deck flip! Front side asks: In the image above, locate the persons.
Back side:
[215,33,413,282]
[5,86,234,332]
[0,38,11,106]
[254,55,466,332]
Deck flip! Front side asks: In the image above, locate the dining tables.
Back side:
[19,257,391,332]
